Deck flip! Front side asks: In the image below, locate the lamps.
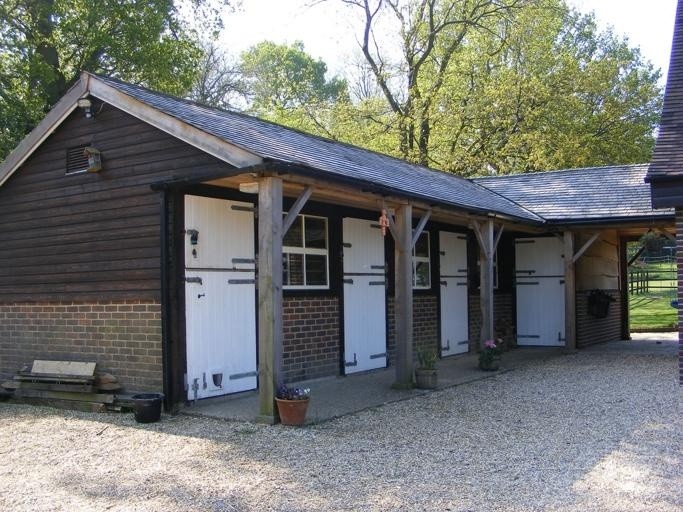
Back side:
[77,96,98,120]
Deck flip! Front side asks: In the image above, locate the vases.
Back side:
[274,396,309,427]
[482,357,501,371]
[130,392,166,423]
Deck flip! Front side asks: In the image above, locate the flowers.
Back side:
[477,338,505,362]
[276,384,312,400]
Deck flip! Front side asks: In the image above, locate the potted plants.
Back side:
[414,344,441,389]
